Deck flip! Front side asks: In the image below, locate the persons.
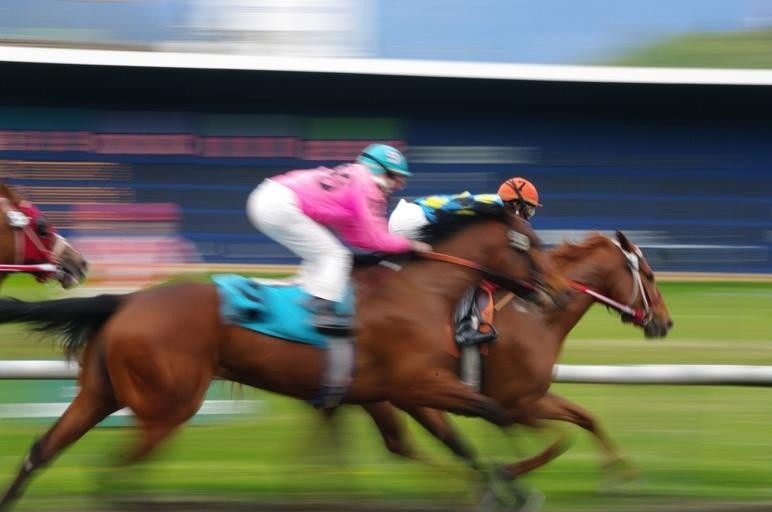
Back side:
[243,142,436,336]
[387,176,544,347]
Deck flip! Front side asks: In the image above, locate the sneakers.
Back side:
[455,316,496,346]
[310,308,357,330]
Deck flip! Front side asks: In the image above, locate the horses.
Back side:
[0,199,675,511]
[0,179,90,290]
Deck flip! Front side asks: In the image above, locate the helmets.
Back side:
[498,177,542,208]
[356,144,412,178]
